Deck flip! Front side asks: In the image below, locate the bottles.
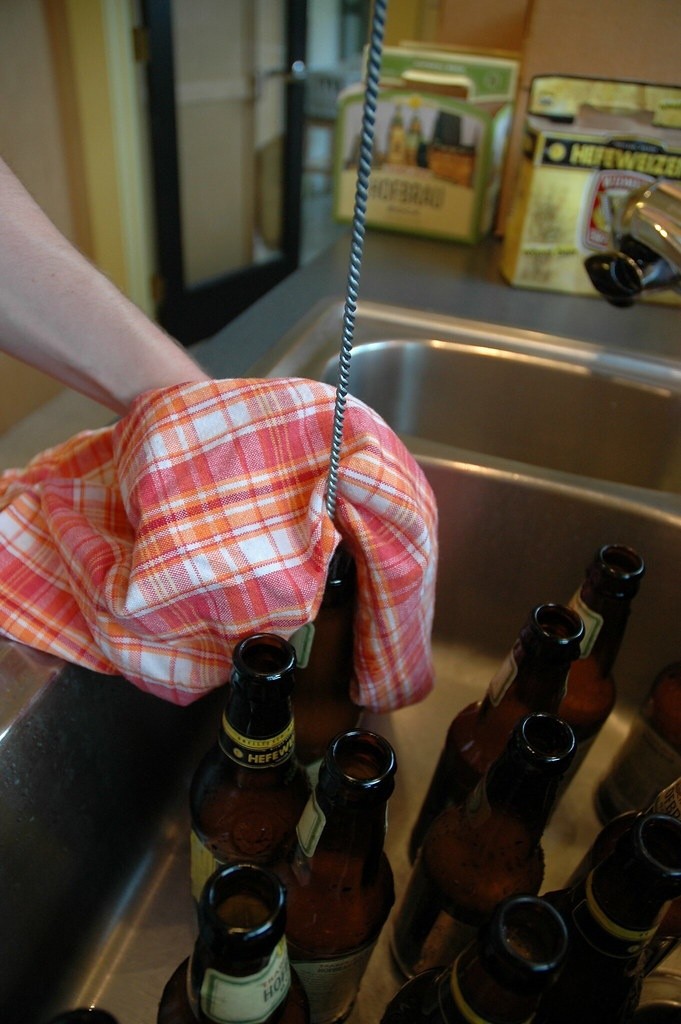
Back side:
[384,104,405,163]
[406,117,420,166]
[41,542,681,1024]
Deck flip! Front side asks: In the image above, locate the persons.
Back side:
[0,158,215,416]
